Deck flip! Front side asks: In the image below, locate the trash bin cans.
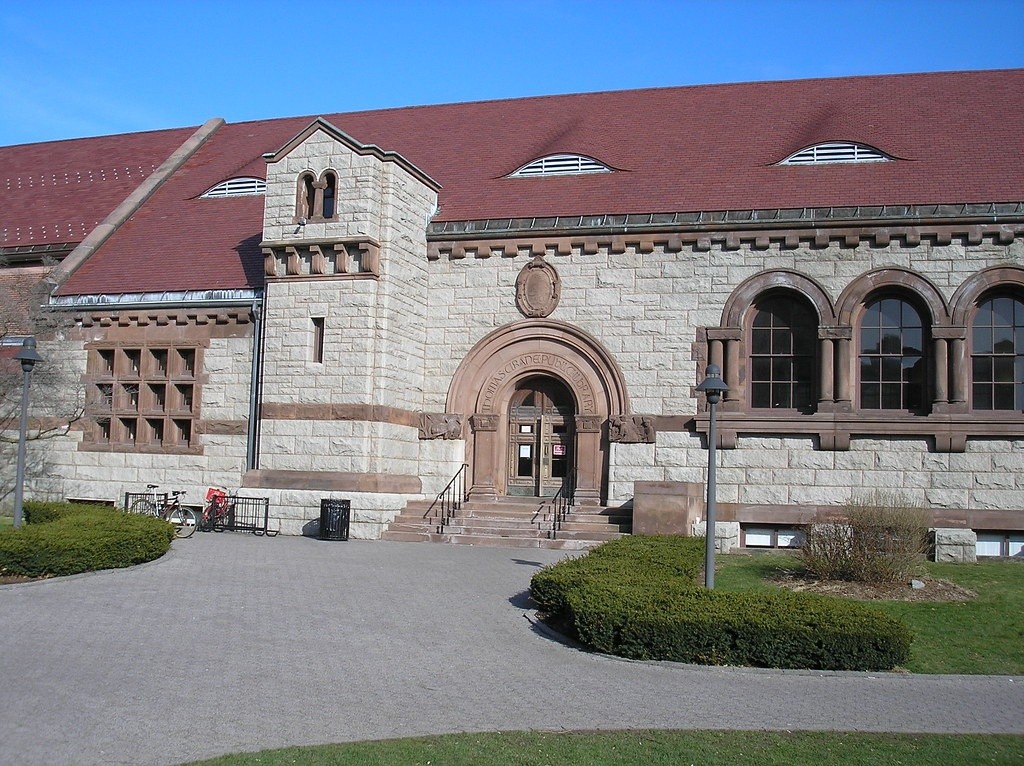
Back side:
[318,497,350,541]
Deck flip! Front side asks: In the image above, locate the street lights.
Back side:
[11,338,44,527]
[695,364,732,588]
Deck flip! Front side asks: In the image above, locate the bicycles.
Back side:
[198,485,242,531]
[132,484,198,539]
